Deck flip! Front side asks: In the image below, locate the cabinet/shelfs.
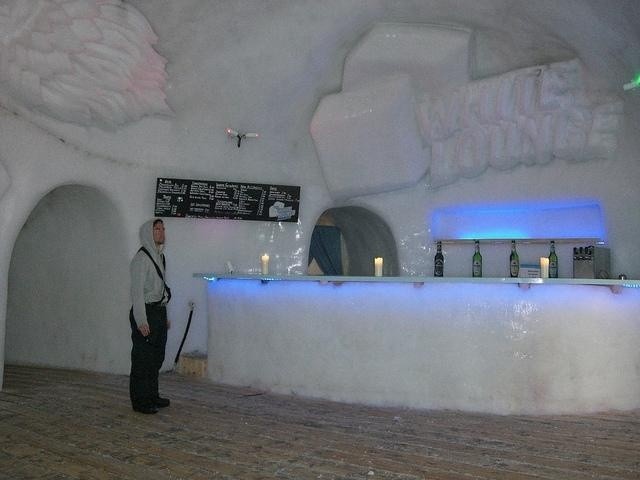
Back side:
[431,240,444,277]
[509,240,520,278]
[548,241,559,279]
[473,240,483,278]
[571,247,591,260]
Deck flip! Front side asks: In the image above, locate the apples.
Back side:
[374,255,384,278]
[261,253,270,275]
[540,256,550,279]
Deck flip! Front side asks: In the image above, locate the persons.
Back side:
[130,218,171,414]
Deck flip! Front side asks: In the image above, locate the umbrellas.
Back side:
[133,396,171,414]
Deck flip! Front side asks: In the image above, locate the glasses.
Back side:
[226,126,259,148]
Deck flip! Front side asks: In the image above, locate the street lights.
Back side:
[163,284,171,304]
